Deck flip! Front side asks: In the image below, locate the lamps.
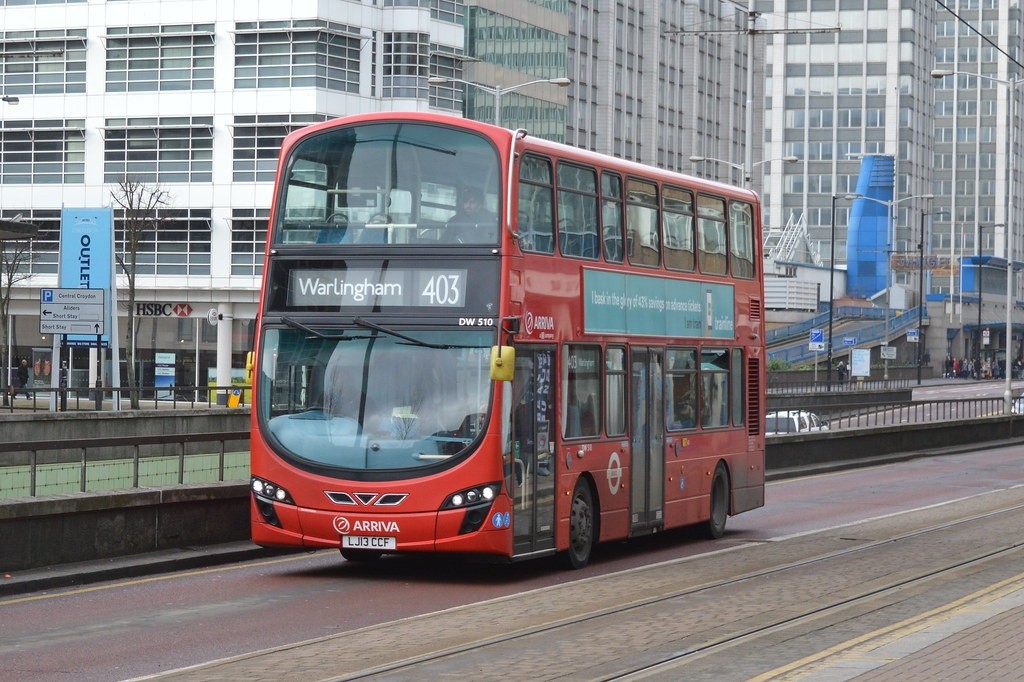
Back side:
[10,212,23,222]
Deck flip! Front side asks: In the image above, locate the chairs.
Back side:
[491,209,680,262]
[316,213,352,244]
[356,212,395,244]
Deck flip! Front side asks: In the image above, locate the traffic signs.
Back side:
[39,289,105,335]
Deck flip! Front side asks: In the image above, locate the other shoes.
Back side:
[27,397,32,400]
[13,396,17,399]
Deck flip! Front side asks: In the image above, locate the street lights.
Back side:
[931,68,1023,417]
[976,223,1005,380]
[828,194,870,392]
[687,155,799,190]
[844,194,935,388]
[428,74,571,129]
[917,210,951,386]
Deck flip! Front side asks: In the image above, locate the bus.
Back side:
[243,111,767,569]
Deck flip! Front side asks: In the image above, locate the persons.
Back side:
[317,366,380,424]
[441,189,496,244]
[568,378,582,438]
[945,355,1002,380]
[837,361,845,386]
[13,359,33,400]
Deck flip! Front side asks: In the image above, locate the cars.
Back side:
[767,410,830,433]
[1011,392,1024,415]
